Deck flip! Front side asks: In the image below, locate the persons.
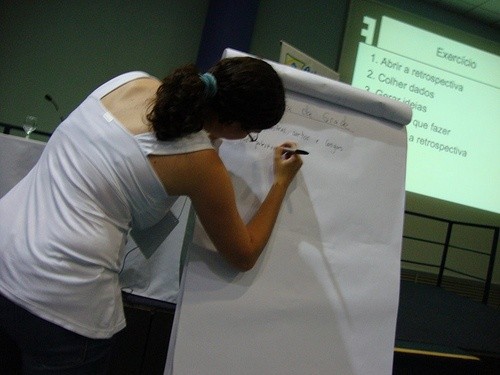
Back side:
[0,56,303,375]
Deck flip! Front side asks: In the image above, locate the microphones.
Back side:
[44,94,65,123]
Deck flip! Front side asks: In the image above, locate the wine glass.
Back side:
[20,115,37,140]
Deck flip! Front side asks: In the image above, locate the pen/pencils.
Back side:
[283,146,310,155]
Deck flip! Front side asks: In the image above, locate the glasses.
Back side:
[247,131,259,142]
